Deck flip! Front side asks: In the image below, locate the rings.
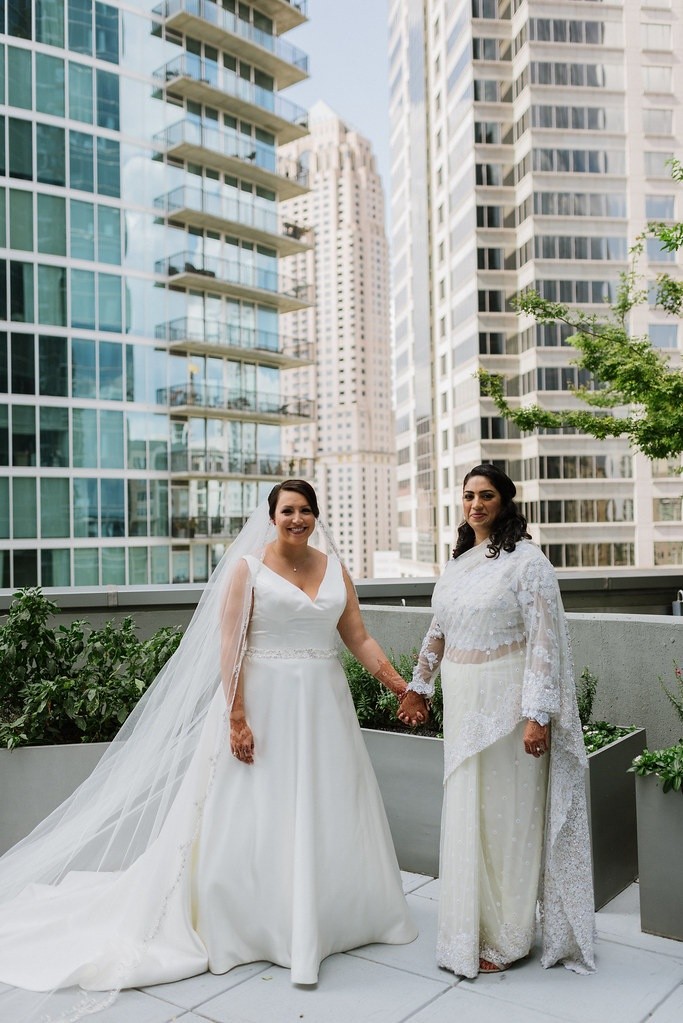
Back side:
[536,747,541,751]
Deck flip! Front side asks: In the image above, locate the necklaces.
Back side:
[270,542,311,571]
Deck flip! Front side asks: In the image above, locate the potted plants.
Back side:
[575,665,647,913]
[626,739,683,942]
[338,647,443,877]
[0,584,184,858]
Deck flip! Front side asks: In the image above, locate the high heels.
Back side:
[479,951,531,973]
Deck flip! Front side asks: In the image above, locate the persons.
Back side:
[396,464,598,979]
[194,479,425,985]
